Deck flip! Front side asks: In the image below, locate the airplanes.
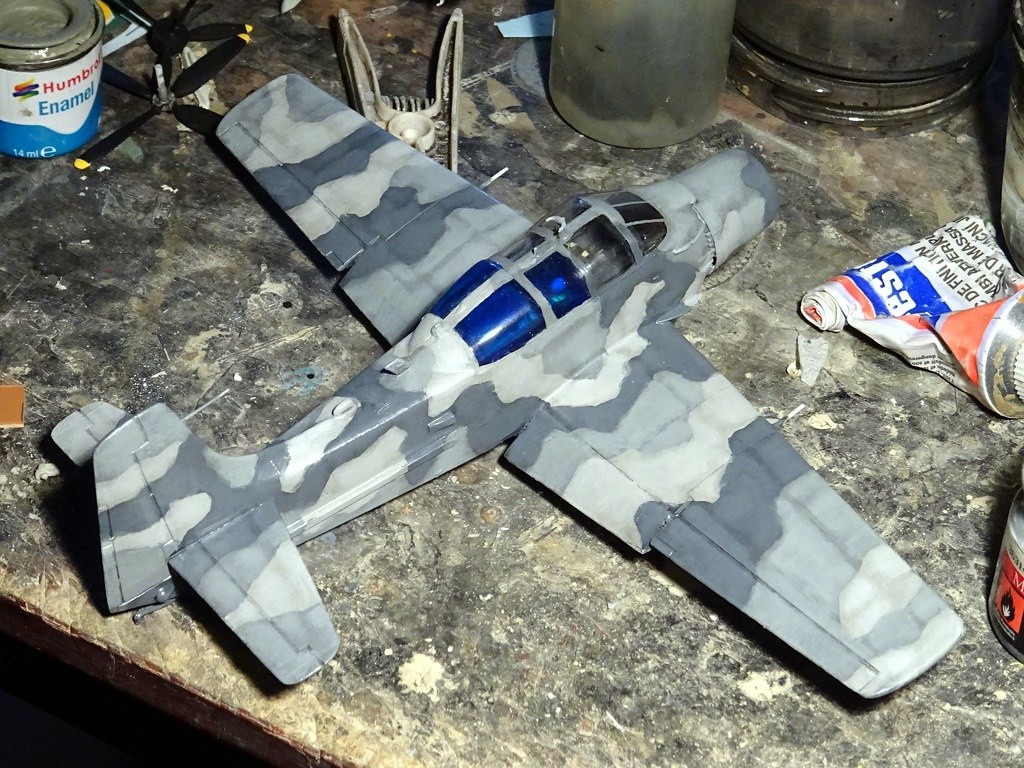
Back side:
[48,70,966,706]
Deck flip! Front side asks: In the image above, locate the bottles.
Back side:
[547,0,736,149]
[986,461,1024,666]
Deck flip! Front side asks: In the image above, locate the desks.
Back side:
[1,0,1024,767]
